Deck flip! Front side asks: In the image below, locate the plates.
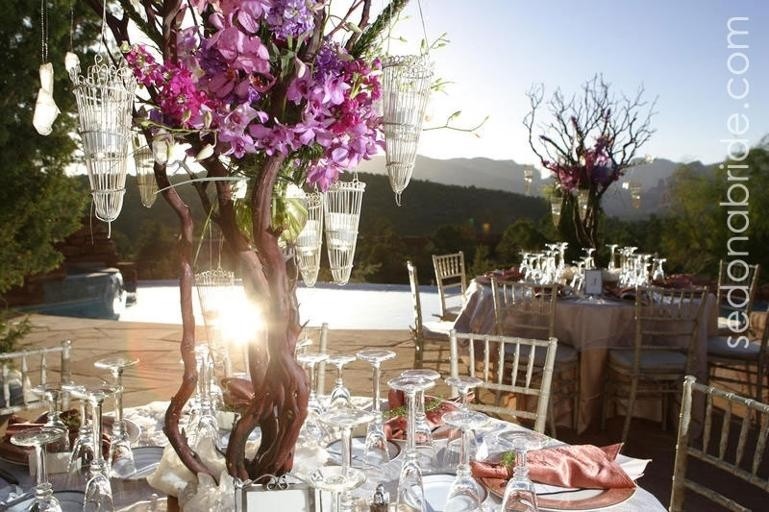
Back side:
[476,445,638,512]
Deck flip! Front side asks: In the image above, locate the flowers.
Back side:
[520,72,660,267]
[80,0,408,487]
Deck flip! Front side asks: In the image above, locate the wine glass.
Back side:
[0,336,538,512]
[519,239,671,295]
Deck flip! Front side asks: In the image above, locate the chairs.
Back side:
[1,250,768,511]
